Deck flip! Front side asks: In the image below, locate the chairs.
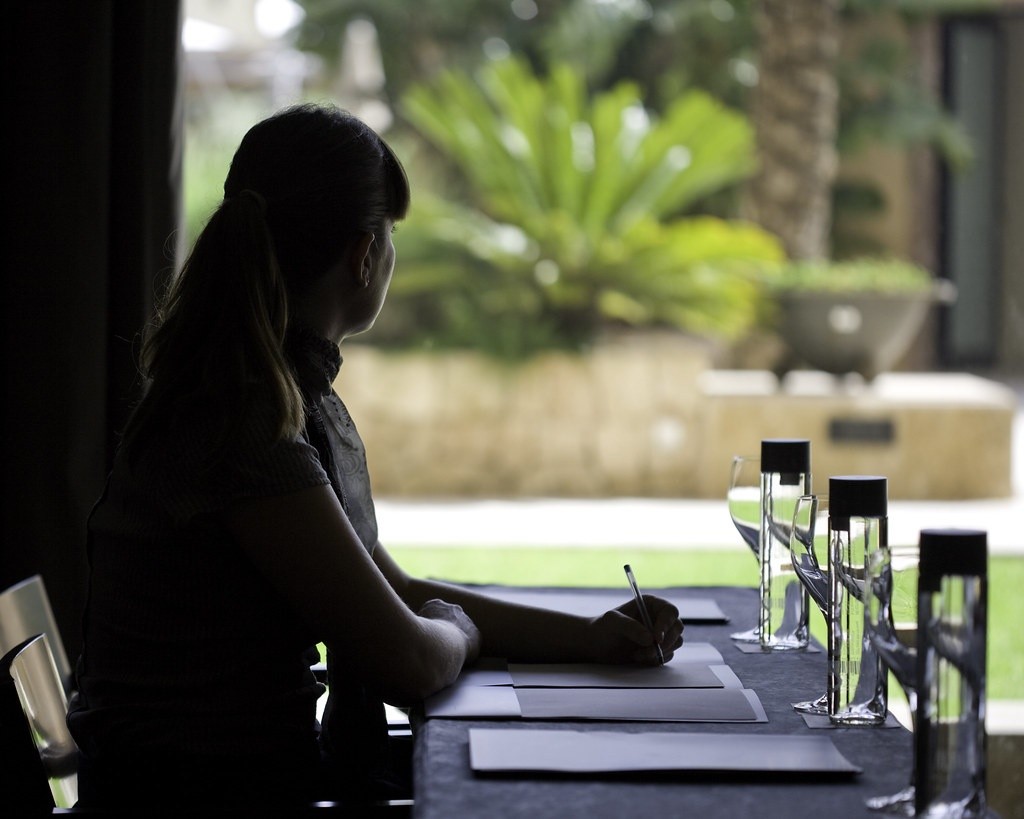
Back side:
[0,575,81,819]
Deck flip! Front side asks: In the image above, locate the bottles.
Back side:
[826,473,890,726]
[759,438,812,651]
[912,529,986,819]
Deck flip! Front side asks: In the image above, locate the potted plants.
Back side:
[759,263,954,383]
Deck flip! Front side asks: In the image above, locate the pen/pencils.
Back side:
[624,564,665,667]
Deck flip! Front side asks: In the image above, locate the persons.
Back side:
[65,102,686,819]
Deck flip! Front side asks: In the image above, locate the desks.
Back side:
[413,584,1008,818]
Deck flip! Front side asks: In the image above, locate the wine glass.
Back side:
[788,495,843,716]
[726,452,762,642]
[860,542,920,816]
